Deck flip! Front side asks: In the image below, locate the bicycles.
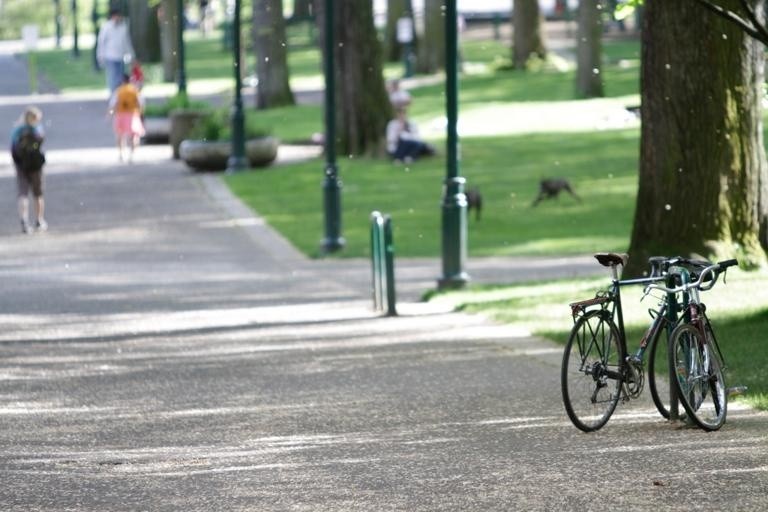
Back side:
[639,257,749,432]
[557,250,714,433]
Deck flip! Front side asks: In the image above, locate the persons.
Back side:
[104,72,147,168]
[386,77,413,133]
[94,9,140,104]
[9,105,48,234]
[385,107,428,168]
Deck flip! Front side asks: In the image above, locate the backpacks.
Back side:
[12,127,44,171]
